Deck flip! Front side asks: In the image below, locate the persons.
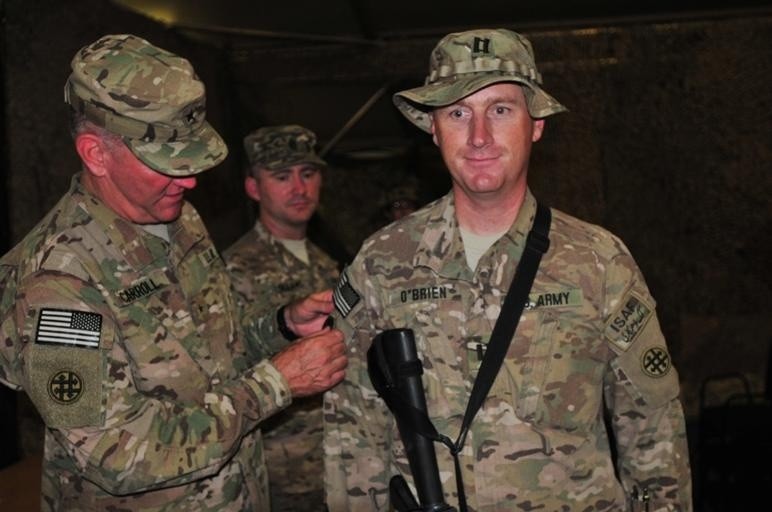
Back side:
[217,125,343,511]
[320,26,695,512]
[379,187,418,222]
[0,34,352,512]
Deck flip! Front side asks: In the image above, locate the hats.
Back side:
[65,35,228,178]
[244,123,329,175]
[392,28,569,133]
